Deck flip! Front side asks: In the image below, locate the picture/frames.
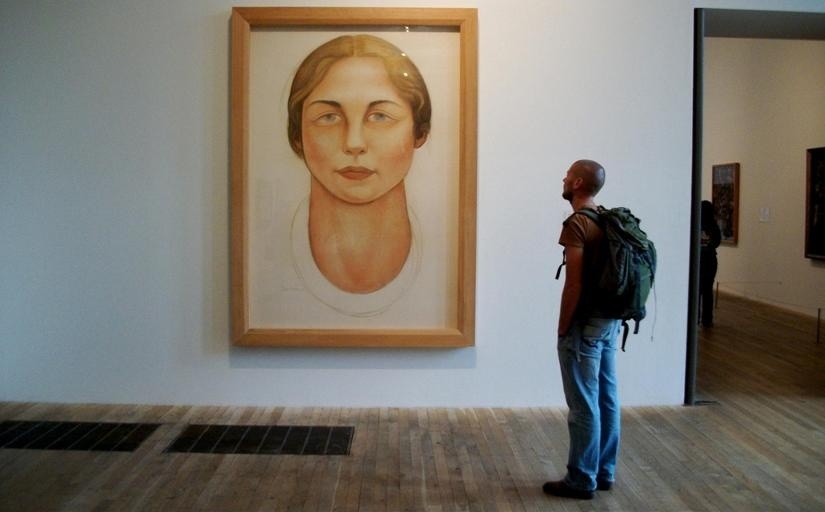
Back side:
[712,164,740,245]
[804,147,824,260]
[230,8,478,346]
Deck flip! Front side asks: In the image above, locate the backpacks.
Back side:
[562,205,657,322]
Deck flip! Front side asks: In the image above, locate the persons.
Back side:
[542,159,622,500]
[699,199,722,332]
[246,31,455,334]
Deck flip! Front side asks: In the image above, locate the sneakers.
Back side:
[542,472,613,498]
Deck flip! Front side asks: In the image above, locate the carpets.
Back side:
[162,424,354,454]
[2,420,164,454]
